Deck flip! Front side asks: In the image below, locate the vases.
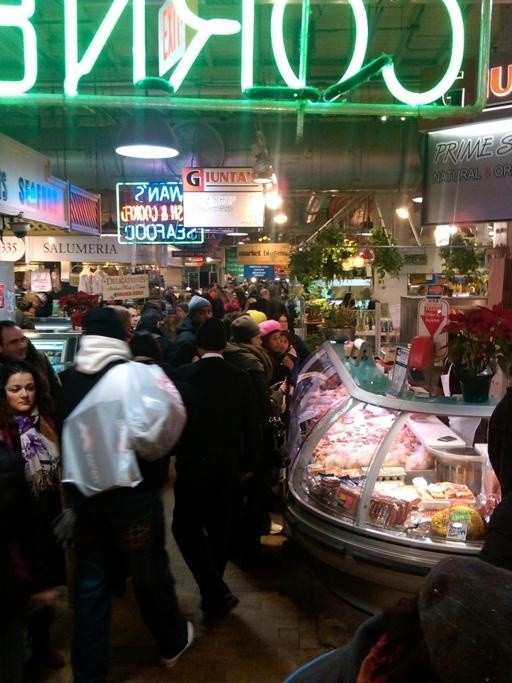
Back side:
[69,306,93,330]
[453,365,497,403]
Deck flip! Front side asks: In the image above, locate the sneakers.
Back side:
[200,593,240,628]
[159,621,195,669]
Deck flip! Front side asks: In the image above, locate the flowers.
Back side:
[440,306,512,375]
[57,290,99,317]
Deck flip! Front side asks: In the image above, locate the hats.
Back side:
[196,316,228,352]
[229,315,262,344]
[258,319,282,339]
[246,309,266,325]
[188,295,212,316]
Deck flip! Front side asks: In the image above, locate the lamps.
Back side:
[250,151,273,184]
[354,196,376,262]
[112,88,181,159]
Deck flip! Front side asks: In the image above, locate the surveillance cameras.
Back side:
[8,222,30,238]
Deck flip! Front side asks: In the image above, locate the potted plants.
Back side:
[322,309,356,342]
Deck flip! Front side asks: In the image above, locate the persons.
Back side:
[16,289,53,316]
[1,355,69,682]
[1,319,63,410]
[228,314,285,536]
[120,275,308,372]
[171,318,252,629]
[478,346,510,567]
[57,306,197,682]
[279,554,511,682]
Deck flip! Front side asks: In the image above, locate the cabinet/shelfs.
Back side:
[284,337,502,618]
[20,316,82,373]
